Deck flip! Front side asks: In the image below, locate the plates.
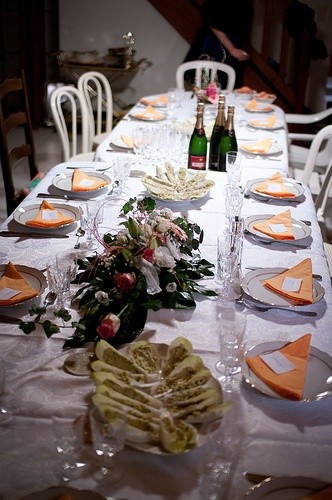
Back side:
[129,110,166,121]
[244,213,311,242]
[244,475,332,500]
[241,340,332,401]
[244,101,274,113]
[240,266,325,307]
[141,98,169,107]
[96,340,224,457]
[0,263,48,307]
[12,203,83,228]
[246,177,305,200]
[247,117,285,130]
[240,140,282,155]
[110,134,148,149]
[52,171,111,193]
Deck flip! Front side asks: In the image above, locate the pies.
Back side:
[90,337,234,457]
[142,162,214,201]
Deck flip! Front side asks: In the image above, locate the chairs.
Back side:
[176,61,236,92]
[0,70,113,221]
[286,107,332,227]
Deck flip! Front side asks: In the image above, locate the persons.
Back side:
[279,0,317,95]
[180,0,255,92]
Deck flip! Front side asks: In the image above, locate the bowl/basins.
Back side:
[70,46,137,65]
[232,87,256,99]
[253,94,277,103]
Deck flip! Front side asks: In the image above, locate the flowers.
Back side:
[19,197,217,345]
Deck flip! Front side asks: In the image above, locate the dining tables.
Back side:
[0,87,332,500]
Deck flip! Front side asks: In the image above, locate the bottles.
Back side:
[188,96,238,172]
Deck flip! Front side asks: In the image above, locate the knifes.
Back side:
[245,266,322,280]
[242,471,271,482]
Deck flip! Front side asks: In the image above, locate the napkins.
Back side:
[0,87,312,397]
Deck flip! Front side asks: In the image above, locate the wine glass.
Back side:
[131,86,195,152]
[209,83,251,151]
[0,151,247,500]
